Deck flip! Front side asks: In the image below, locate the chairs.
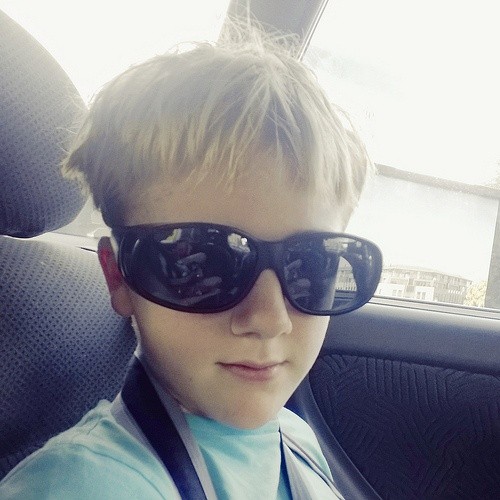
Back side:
[0,10,142,481]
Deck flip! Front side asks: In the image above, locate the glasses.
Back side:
[111,223,382,316]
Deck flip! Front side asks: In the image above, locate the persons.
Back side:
[0,15,385,500]
